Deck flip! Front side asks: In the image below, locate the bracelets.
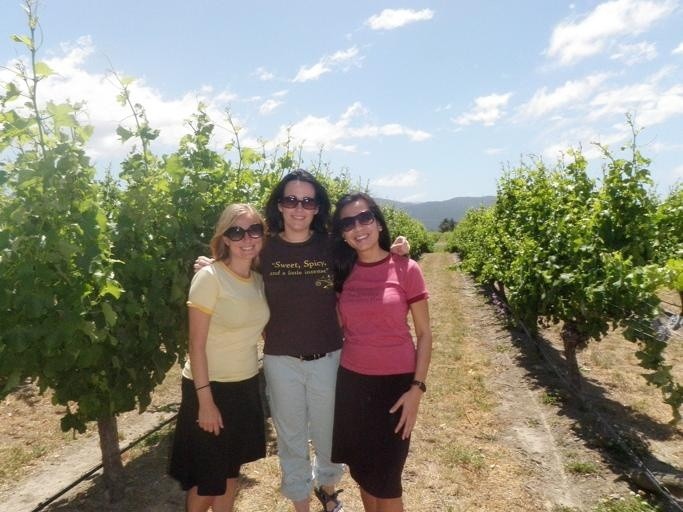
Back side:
[411,381,426,392]
[192,384,210,393]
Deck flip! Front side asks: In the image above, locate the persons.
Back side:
[194,168,410,512]
[330,190,432,512]
[170,203,271,512]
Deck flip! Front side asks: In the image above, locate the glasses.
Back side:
[278,195,318,210]
[337,211,376,233]
[223,224,264,242]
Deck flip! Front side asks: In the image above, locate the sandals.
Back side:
[315,484,345,511]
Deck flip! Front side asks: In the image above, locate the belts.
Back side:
[291,351,326,360]
